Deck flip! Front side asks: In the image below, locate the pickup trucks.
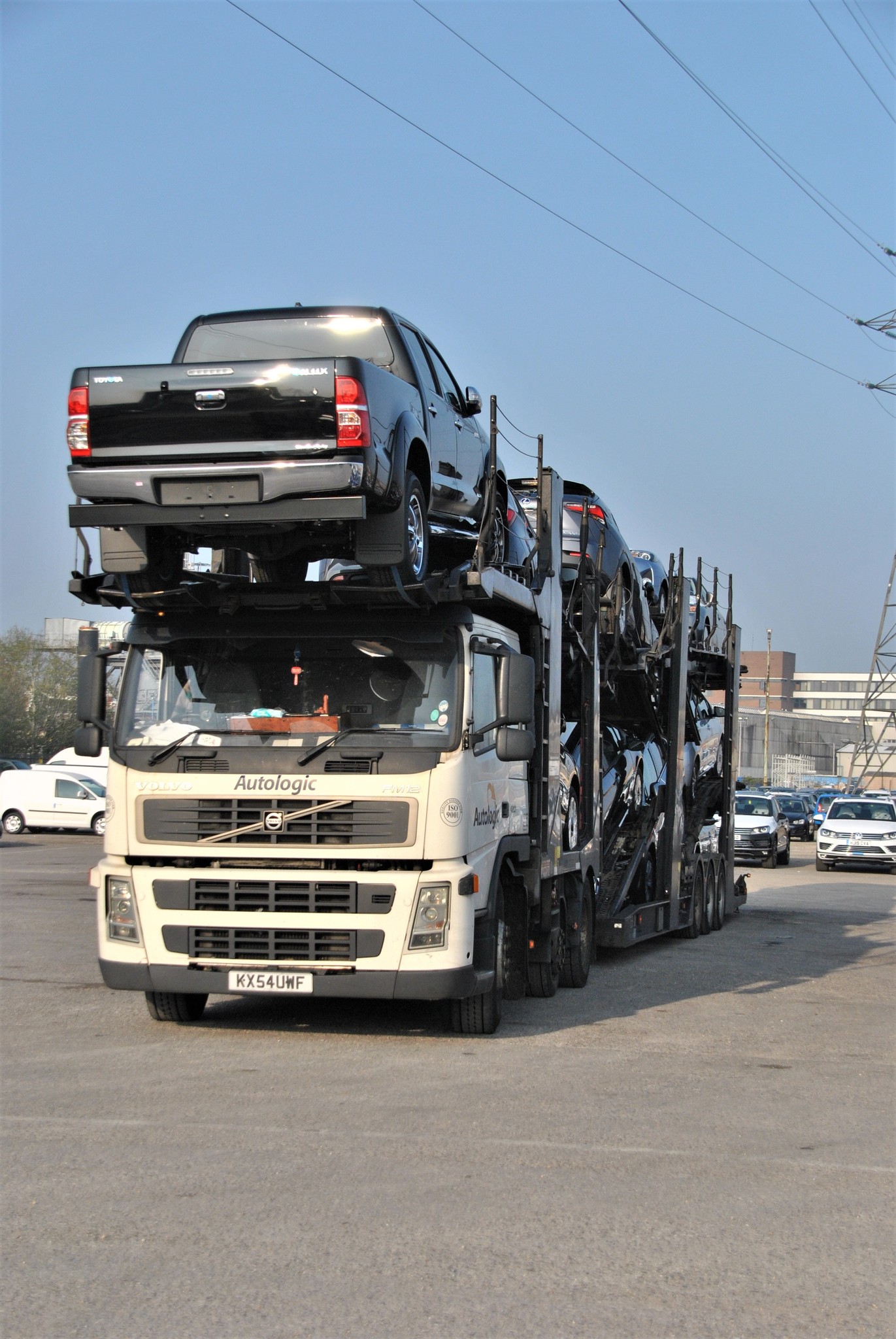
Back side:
[64,303,509,584]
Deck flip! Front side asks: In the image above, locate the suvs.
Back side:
[816,797,896,874]
[734,789,792,870]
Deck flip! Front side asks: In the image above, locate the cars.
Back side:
[0,745,108,836]
[555,686,724,849]
[735,787,896,877]
[509,469,727,646]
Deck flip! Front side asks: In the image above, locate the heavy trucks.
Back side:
[74,396,750,1039]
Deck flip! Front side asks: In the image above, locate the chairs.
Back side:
[202,661,263,714]
[794,802,802,809]
[838,807,855,818]
